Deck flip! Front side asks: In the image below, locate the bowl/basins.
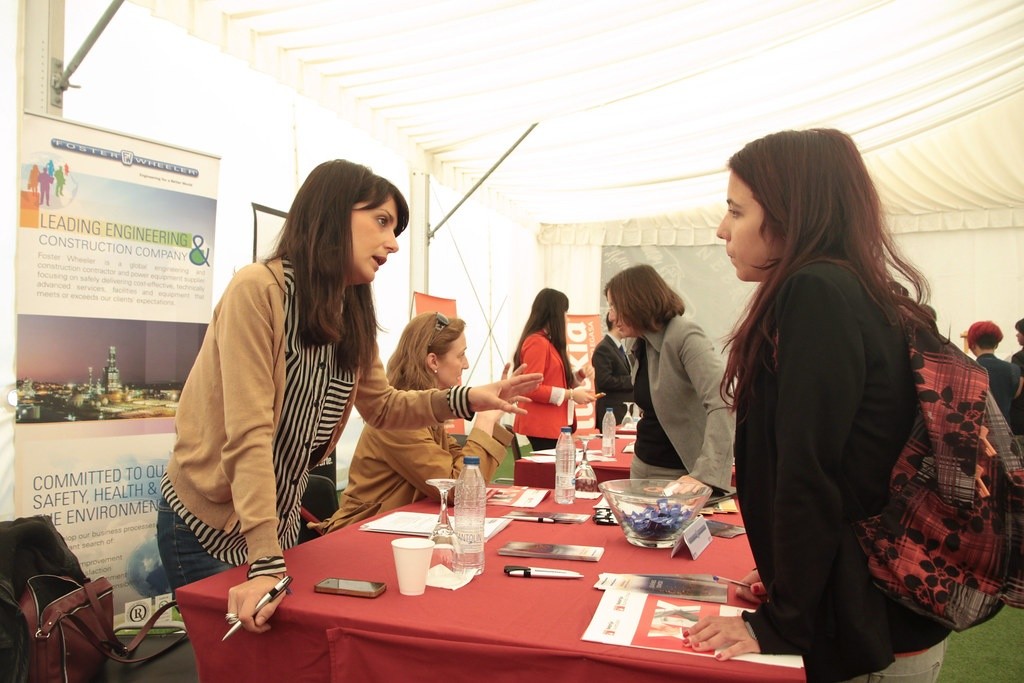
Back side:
[597,479,713,550]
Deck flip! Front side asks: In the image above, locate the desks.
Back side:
[176,481,807,683]
[514,426,736,488]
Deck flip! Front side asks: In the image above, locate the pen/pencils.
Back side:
[500,516,554,522]
[503,565,580,574]
[712,576,751,588]
[509,569,585,579]
[221,576,294,642]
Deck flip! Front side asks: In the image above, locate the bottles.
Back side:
[452,455,487,575]
[602,408,616,458]
[554,426,577,504]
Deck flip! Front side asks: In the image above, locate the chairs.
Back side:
[0,521,198,683]
[297,473,339,546]
[452,423,533,485]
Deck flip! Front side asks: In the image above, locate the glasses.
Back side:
[428,312,449,347]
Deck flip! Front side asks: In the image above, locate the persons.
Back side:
[307,313,532,534]
[592,312,635,434]
[967,320,1019,429]
[681,129,952,683]
[1011,318,1024,434]
[512,287,598,452]
[603,264,735,496]
[157,159,543,595]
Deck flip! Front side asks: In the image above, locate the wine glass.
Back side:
[621,402,635,429]
[426,478,465,587]
[576,435,598,502]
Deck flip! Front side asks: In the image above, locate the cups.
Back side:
[390,537,436,597]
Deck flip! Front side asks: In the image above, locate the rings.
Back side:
[226,613,240,624]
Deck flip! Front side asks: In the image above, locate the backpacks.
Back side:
[783,257,1024,632]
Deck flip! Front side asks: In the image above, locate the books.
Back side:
[498,541,604,562]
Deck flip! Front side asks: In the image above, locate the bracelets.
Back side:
[578,368,586,379]
[570,389,573,400]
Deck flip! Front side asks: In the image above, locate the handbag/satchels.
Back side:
[20,574,113,683]
